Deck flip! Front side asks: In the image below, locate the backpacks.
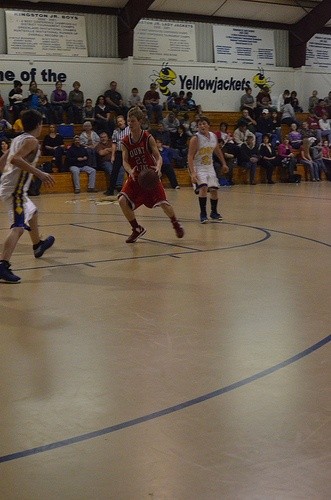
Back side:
[40,161,55,173]
[220,175,231,185]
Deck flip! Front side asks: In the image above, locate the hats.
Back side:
[73,135,80,141]
[262,109,269,114]
[247,132,254,136]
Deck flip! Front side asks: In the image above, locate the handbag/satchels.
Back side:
[287,173,301,183]
[279,157,292,168]
[28,178,42,196]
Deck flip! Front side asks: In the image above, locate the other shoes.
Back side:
[175,186,180,189]
[88,187,99,192]
[103,190,113,195]
[268,179,275,184]
[75,189,80,193]
[250,180,256,185]
[313,177,319,182]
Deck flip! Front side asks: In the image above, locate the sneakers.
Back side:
[126,225,146,243]
[0,261,23,283]
[172,220,184,238]
[33,236,55,258]
[200,213,208,223]
[210,212,223,220]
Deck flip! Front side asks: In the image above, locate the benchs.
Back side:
[38,111,317,194]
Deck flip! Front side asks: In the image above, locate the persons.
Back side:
[118,106,185,242]
[188,117,229,224]
[0,80,331,196]
[0,109,57,284]
